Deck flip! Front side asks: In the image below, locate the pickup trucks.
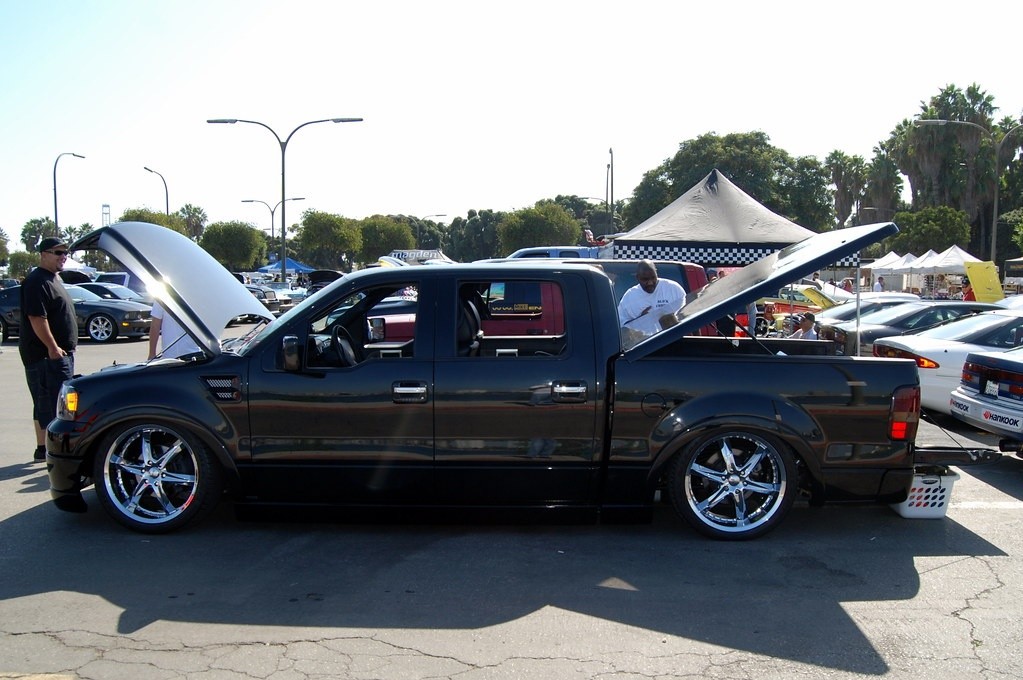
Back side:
[42,220,922,540]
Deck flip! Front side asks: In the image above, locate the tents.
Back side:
[595,169,861,358]
[857,244,1001,300]
[259,257,317,287]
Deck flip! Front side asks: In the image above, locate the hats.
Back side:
[962,279,970,288]
[798,313,815,322]
[40,237,69,252]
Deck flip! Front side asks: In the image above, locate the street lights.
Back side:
[914,119,1022,265]
[142,167,170,217]
[53,152,87,236]
[573,194,639,234]
[392,214,447,249]
[241,197,306,254]
[205,116,365,279]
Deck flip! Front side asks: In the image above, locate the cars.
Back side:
[0,242,719,345]
[754,280,1023,458]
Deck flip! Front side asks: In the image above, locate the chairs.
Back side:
[457,299,485,357]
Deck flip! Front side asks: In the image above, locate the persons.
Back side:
[812,273,854,293]
[527,438,557,460]
[274,274,281,282]
[873,276,885,292]
[961,279,977,302]
[525,377,559,407]
[148,298,205,362]
[817,325,835,340]
[621,294,686,337]
[616,258,686,325]
[718,270,727,280]
[747,300,759,337]
[296,273,308,288]
[864,278,870,286]
[932,274,948,293]
[777,312,818,339]
[948,287,963,300]
[19,238,78,463]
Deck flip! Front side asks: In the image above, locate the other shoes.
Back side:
[34,449,46,462]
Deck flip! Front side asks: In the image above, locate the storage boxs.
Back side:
[889,466,960,519]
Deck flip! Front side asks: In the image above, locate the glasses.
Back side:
[43,250,68,256]
[801,319,807,322]
